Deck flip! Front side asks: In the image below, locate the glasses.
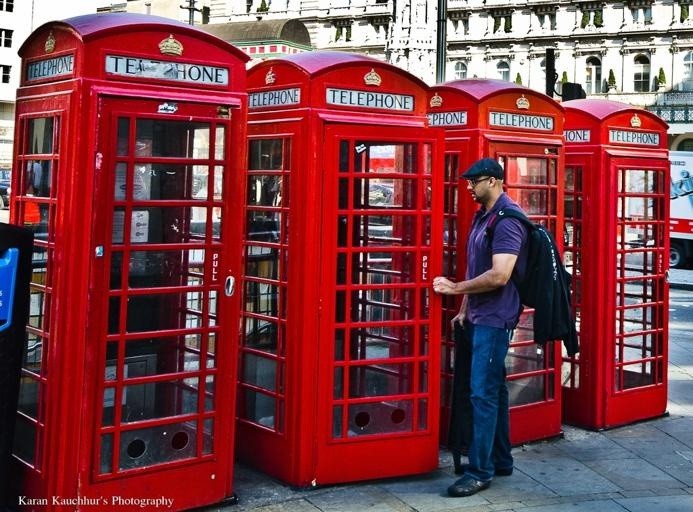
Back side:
[468,177,490,185]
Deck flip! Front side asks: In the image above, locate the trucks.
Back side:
[616,151,693,266]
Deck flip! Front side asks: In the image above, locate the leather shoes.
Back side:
[494,467,513,476]
[447,474,490,497]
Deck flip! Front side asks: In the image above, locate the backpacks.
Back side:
[484,207,552,315]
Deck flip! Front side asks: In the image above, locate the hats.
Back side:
[461,157,505,179]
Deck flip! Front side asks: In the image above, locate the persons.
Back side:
[432,155,530,498]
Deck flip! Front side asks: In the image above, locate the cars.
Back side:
[0,166,11,210]
